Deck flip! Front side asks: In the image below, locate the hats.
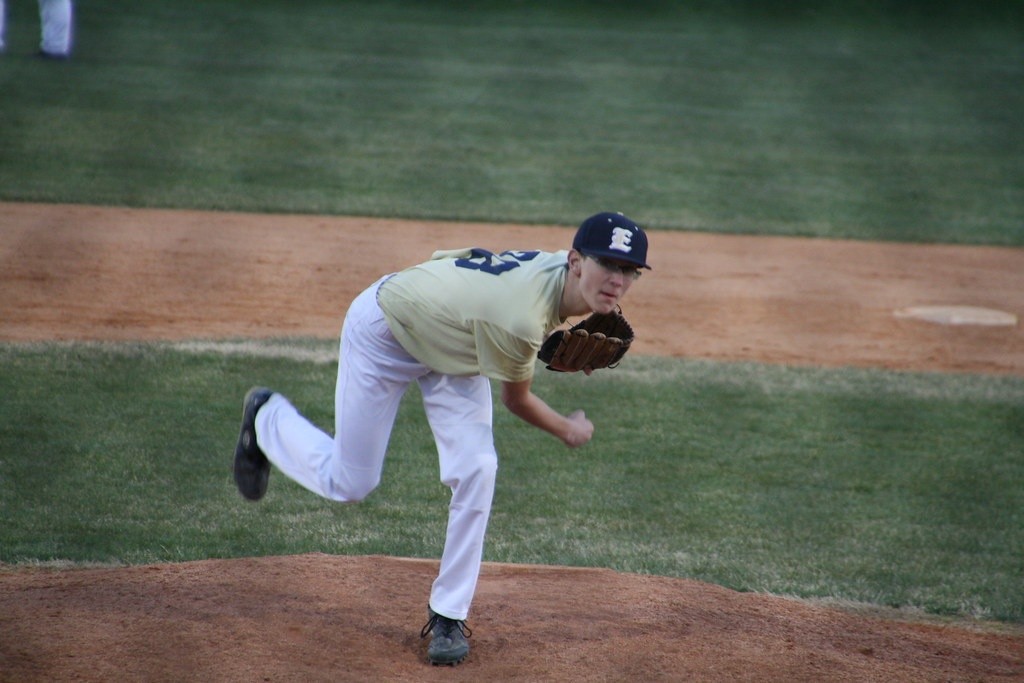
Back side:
[572,213,650,270]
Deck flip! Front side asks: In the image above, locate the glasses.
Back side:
[581,251,641,282]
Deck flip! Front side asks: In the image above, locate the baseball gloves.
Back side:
[535,301,637,377]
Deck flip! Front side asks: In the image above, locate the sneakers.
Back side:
[420,605,473,665]
[234,385,274,501]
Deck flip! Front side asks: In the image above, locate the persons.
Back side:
[232,210,652,669]
[0,0,73,60]
